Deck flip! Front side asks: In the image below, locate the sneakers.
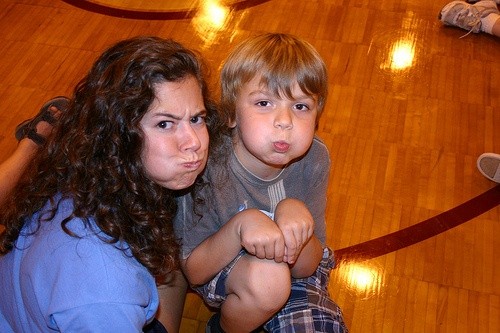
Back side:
[439,0,498,35]
[477,153,500,183]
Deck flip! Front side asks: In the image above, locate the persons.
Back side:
[169,23,350,332]
[440,0,500,41]
[2,33,234,333]
[0,90,71,217]
